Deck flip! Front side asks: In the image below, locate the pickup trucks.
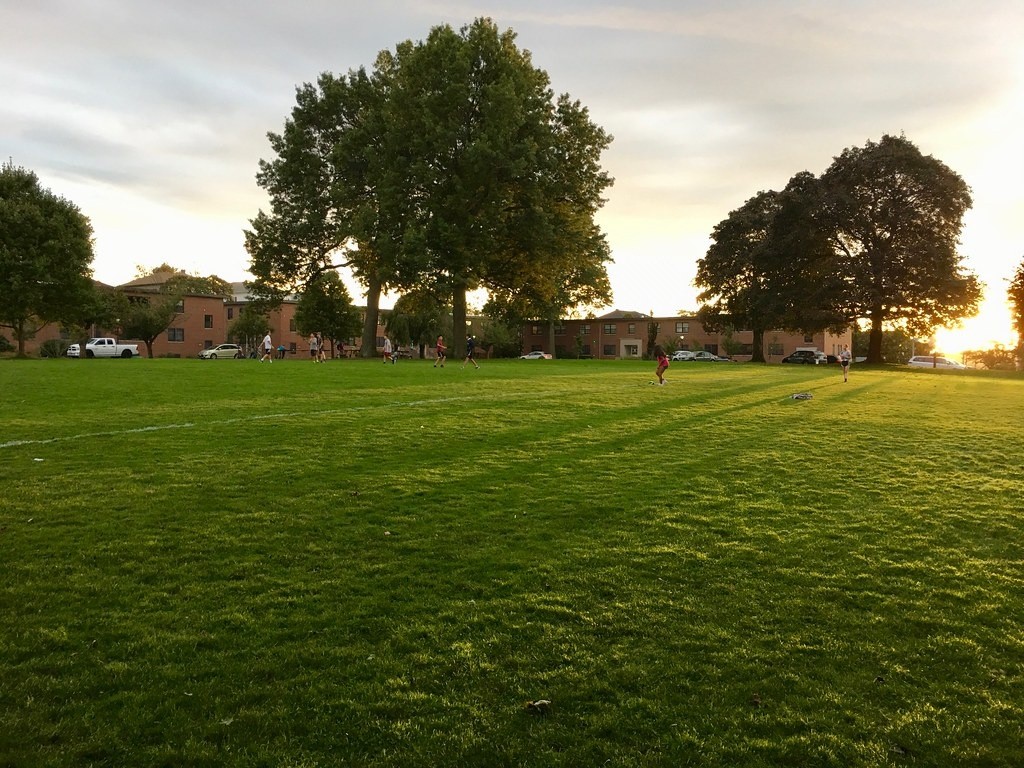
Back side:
[519,351,552,360]
[66,338,139,359]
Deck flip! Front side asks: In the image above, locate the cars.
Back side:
[782,350,828,365]
[825,354,843,363]
[198,343,244,359]
[684,351,718,362]
[711,357,738,362]
[666,351,697,362]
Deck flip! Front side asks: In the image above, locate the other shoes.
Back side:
[434,365,436,368]
[392,360,396,364]
[476,367,480,369]
[460,367,464,369]
[663,380,667,384]
[440,365,444,368]
[259,359,263,364]
[659,383,663,386]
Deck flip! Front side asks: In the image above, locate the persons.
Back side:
[840,343,852,383]
[434,334,448,367]
[655,350,670,385]
[459,334,481,370]
[316,331,327,364]
[238,343,288,359]
[335,339,415,359]
[306,333,320,363]
[380,334,395,363]
[257,329,273,364]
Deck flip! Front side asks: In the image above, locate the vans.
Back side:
[907,356,966,370]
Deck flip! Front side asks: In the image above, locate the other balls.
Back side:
[648,381,655,385]
